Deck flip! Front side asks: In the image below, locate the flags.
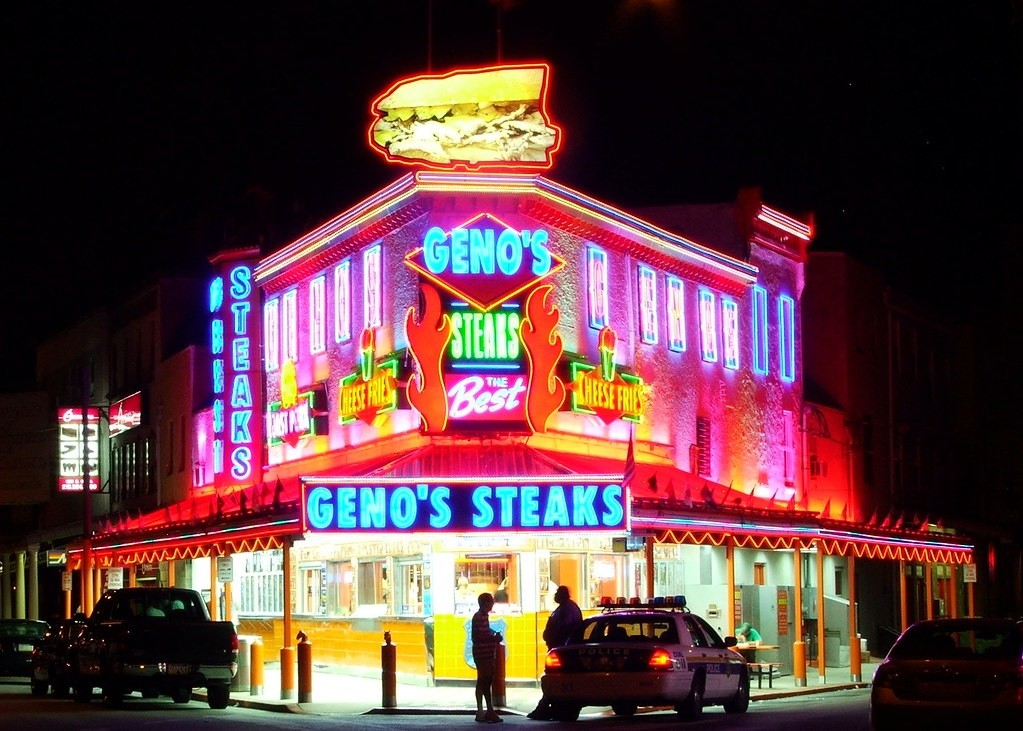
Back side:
[648,476,943,533]
[93,481,284,535]
[621,428,635,490]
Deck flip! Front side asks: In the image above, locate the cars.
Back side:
[0,619,53,675]
[541,597,752,723]
[870,616,1023,731]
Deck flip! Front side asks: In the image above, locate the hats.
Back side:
[740,623,752,634]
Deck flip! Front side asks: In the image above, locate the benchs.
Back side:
[747,663,784,690]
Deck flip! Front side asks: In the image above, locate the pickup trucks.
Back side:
[31,587,240,711]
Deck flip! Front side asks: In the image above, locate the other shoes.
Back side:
[475,710,501,722]
[526,705,560,720]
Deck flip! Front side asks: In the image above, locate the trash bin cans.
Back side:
[229,635,262,692]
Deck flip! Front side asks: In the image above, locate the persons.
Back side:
[526,586,583,720]
[471,593,504,722]
[735,623,763,679]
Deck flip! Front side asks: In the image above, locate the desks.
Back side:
[739,644,782,649]
